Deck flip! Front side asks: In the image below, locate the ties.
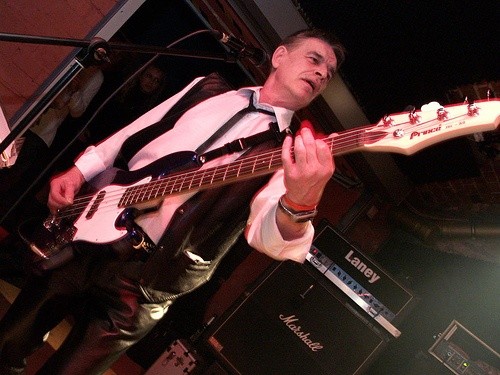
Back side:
[195,94,275,154]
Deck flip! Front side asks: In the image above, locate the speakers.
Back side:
[195,260,391,375]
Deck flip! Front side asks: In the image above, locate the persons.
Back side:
[0,30,343,375]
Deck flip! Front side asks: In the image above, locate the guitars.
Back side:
[25,90,500,277]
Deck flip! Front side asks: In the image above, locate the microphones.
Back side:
[212,30,270,69]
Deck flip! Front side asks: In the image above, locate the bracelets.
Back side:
[281,194,319,212]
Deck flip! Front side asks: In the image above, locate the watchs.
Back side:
[278,194,318,224]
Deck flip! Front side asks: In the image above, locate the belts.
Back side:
[124,224,156,253]
